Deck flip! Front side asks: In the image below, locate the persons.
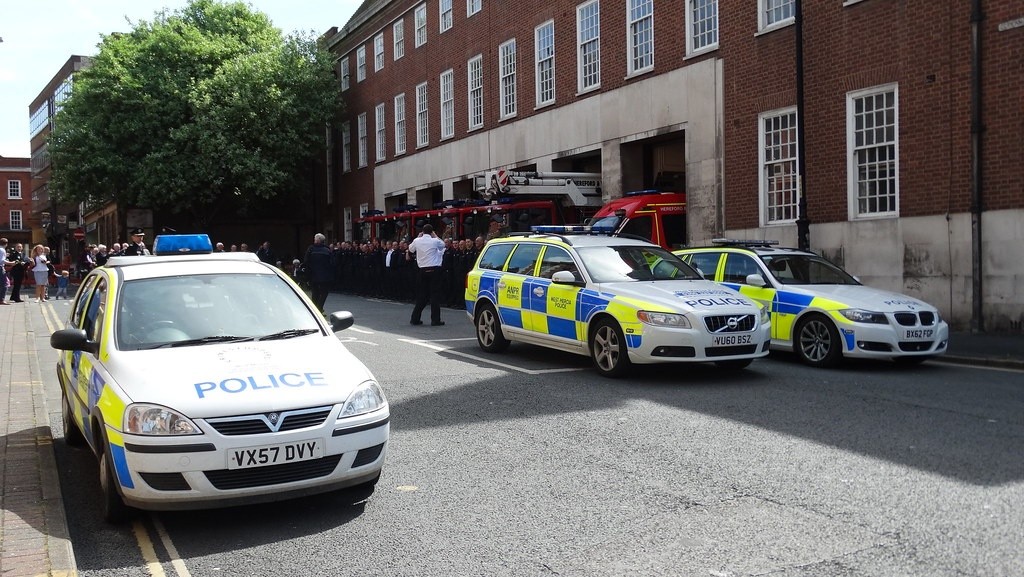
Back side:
[122,282,186,345]
[214,242,249,252]
[11,242,32,302]
[124,227,151,255]
[54,270,70,300]
[50,249,60,264]
[256,241,273,264]
[0,238,18,305]
[304,232,330,314]
[407,224,446,326]
[82,242,130,279]
[330,236,487,310]
[290,259,300,287]
[29,244,55,300]
[622,255,639,270]
[76,280,107,340]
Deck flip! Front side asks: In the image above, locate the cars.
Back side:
[47,231,391,526]
[459,223,773,378]
[648,237,951,369]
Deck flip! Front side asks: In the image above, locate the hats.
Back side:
[129,227,145,236]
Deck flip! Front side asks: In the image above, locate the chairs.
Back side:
[770,258,786,278]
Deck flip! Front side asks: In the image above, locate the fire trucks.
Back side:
[472,170,603,240]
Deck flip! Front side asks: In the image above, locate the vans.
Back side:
[582,189,688,250]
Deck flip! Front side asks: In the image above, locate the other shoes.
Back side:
[10,297,15,301]
[15,299,24,302]
[42,298,47,302]
[321,311,328,317]
[410,320,423,325]
[431,321,445,326]
[35,298,40,302]
[0,301,10,305]
